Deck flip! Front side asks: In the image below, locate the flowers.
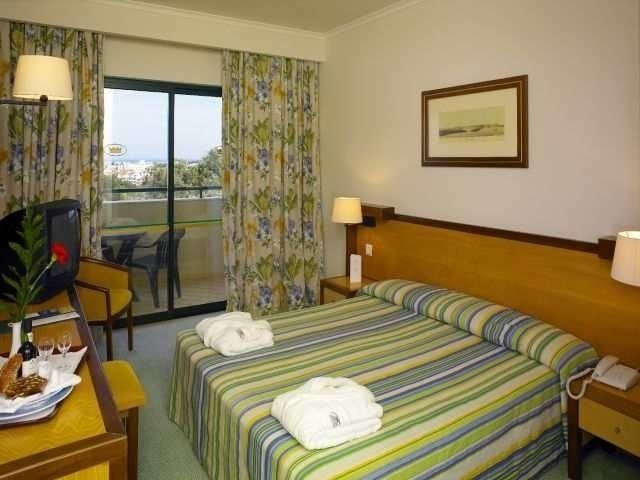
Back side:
[2,199,71,322]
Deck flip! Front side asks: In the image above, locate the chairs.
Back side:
[124,228,185,308]
[75,256,133,361]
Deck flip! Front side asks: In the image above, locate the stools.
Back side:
[99,360,144,480]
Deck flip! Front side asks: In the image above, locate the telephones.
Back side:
[592,355,640,392]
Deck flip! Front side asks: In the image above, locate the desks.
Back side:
[0,286,128,480]
[101,231,148,302]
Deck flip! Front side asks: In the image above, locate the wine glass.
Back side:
[37,336,55,366]
[56,334,72,369]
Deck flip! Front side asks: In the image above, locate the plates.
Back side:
[0,344,90,425]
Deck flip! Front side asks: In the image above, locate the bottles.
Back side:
[17,318,39,381]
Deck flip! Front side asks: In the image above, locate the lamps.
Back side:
[330,196,367,228]
[611,230,640,287]
[0,54,74,106]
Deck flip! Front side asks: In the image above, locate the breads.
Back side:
[4,373,48,400]
[0,353,23,393]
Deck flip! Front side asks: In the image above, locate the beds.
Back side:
[170,279,600,480]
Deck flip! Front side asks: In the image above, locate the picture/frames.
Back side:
[421,75,528,167]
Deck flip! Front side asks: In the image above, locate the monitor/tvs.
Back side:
[0,198,82,305]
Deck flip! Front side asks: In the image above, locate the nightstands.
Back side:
[568,366,640,480]
[320,274,377,304]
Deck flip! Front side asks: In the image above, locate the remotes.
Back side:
[32,311,81,326]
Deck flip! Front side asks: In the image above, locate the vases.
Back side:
[8,320,23,362]
